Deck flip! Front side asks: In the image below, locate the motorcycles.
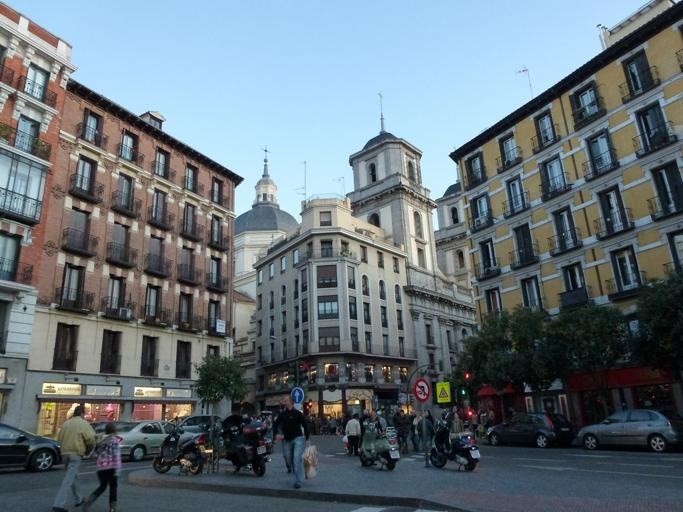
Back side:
[153,415,213,476]
[358,409,400,470]
[426,403,481,472]
[218,411,273,477]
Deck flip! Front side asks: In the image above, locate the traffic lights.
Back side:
[287,361,299,385]
[298,362,308,386]
[308,399,311,408]
[303,402,309,415]
[464,406,475,418]
[464,368,474,381]
[456,385,471,399]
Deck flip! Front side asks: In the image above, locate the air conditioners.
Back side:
[474,216,488,227]
[119,306,132,318]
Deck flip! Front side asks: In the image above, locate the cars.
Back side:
[575,407,682,453]
[96,420,179,462]
[165,415,223,434]
[0,423,62,473]
[486,412,574,448]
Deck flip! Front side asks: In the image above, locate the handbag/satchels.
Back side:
[303,441,321,479]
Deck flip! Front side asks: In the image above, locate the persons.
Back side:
[250,395,495,490]
[52,406,96,512]
[82,422,123,512]
